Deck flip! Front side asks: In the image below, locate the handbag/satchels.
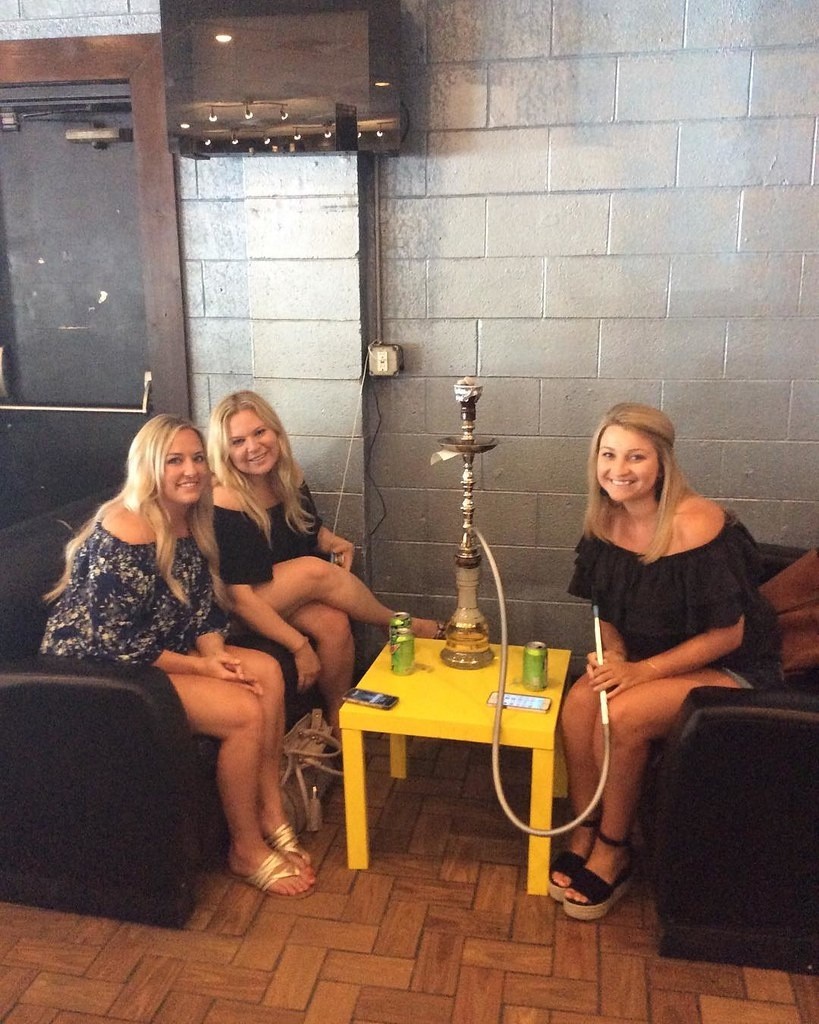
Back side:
[278,709,344,835]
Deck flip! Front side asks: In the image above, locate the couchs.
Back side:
[638,543,819,975]
[0,485,327,928]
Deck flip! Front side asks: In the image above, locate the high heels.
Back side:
[550,819,602,902]
[563,830,640,920]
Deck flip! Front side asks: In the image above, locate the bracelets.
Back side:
[288,636,309,656]
[642,659,662,674]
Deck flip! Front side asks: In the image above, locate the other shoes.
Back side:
[328,746,373,772]
[433,621,449,639]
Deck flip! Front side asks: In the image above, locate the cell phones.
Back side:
[342,688,400,710]
[486,691,551,714]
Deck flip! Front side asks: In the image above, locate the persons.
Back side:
[39,415,317,899]
[206,391,446,780]
[549,403,781,921]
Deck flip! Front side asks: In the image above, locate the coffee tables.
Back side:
[339,638,571,895]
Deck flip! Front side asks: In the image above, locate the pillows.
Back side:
[756,546,819,671]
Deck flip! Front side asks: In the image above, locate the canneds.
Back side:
[389,611,415,675]
[523,641,549,691]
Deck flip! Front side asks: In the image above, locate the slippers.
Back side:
[265,821,311,868]
[229,850,316,899]
